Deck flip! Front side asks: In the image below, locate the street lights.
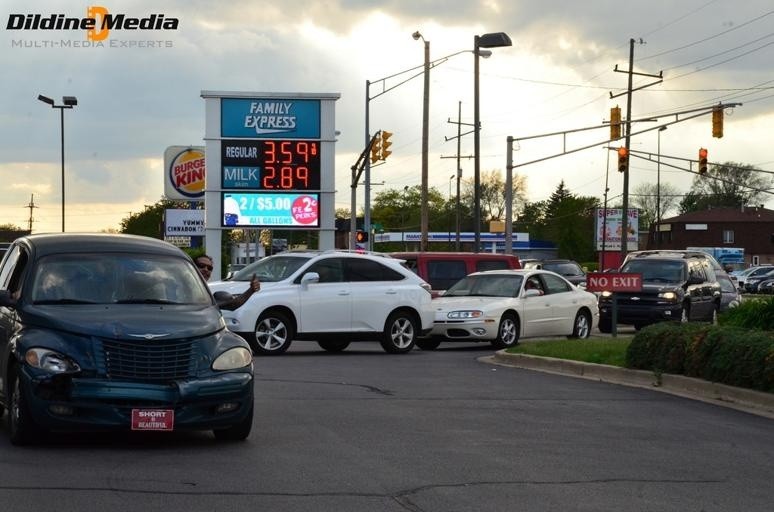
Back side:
[362,48,492,252]
[412,31,430,251]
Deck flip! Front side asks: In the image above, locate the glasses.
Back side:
[197,263,213,272]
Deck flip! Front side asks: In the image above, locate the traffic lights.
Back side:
[698,148,707,175]
[355,231,369,243]
[617,147,628,172]
[369,130,393,164]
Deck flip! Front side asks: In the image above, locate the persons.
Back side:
[192,254,261,312]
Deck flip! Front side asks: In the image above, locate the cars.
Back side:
[415,268,600,351]
[0,233,256,449]
[598,250,773,335]
[523,258,588,290]
[203,248,436,356]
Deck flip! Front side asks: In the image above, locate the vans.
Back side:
[387,251,520,298]
